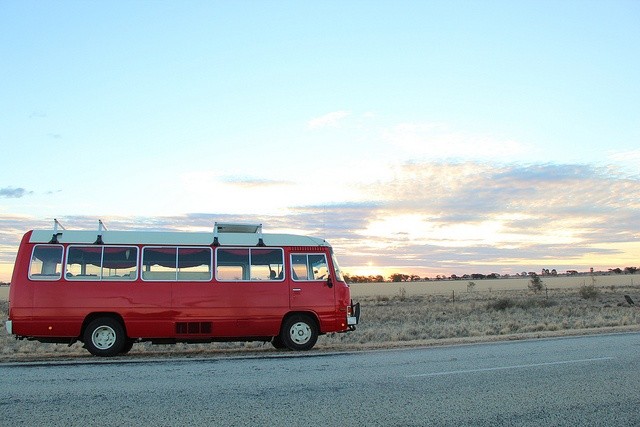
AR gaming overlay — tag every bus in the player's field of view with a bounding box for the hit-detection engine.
[6,218,360,355]
